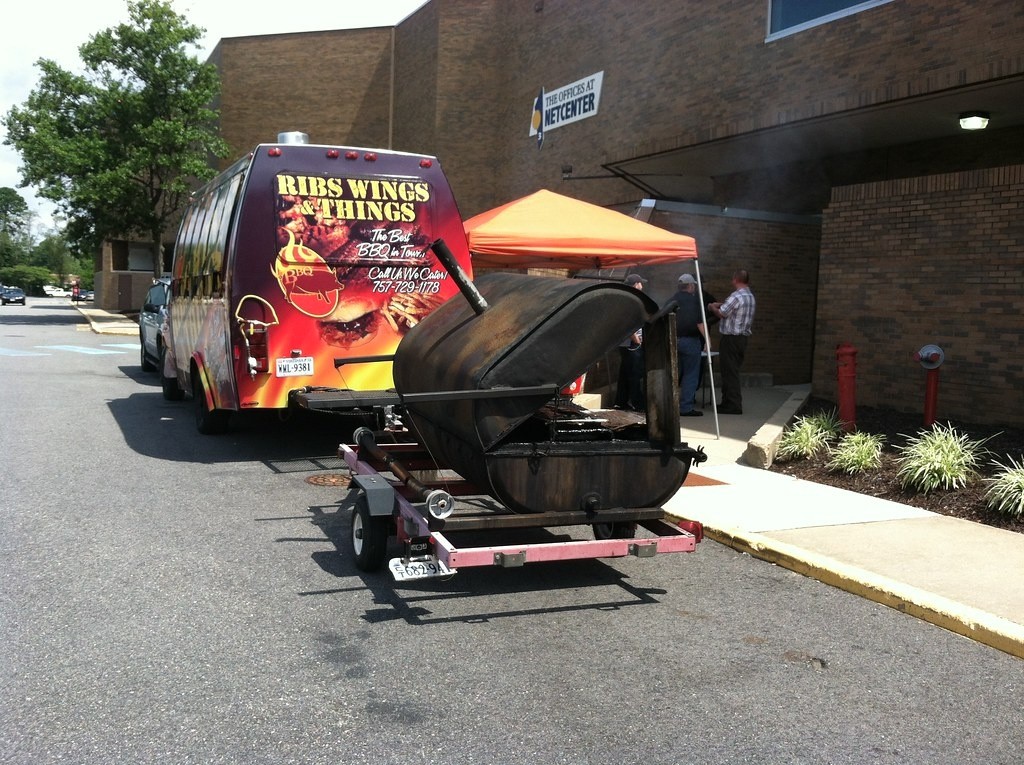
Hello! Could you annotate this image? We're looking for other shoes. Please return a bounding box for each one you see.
[680,410,703,417]
[713,404,742,414]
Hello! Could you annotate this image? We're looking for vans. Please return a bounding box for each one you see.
[162,131,474,437]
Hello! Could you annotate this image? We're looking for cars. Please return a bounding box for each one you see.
[71,289,87,301]
[139,277,182,401]
[86,291,95,301]
[0,288,26,306]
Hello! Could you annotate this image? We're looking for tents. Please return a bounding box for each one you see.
[463,189,721,439]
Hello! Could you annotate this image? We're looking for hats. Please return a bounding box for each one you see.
[624,274,648,286]
[678,273,697,284]
[692,272,707,284]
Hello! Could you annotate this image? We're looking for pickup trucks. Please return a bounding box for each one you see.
[45,288,73,297]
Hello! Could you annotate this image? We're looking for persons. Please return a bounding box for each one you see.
[613,274,649,413]
[707,270,756,415]
[666,272,720,416]
